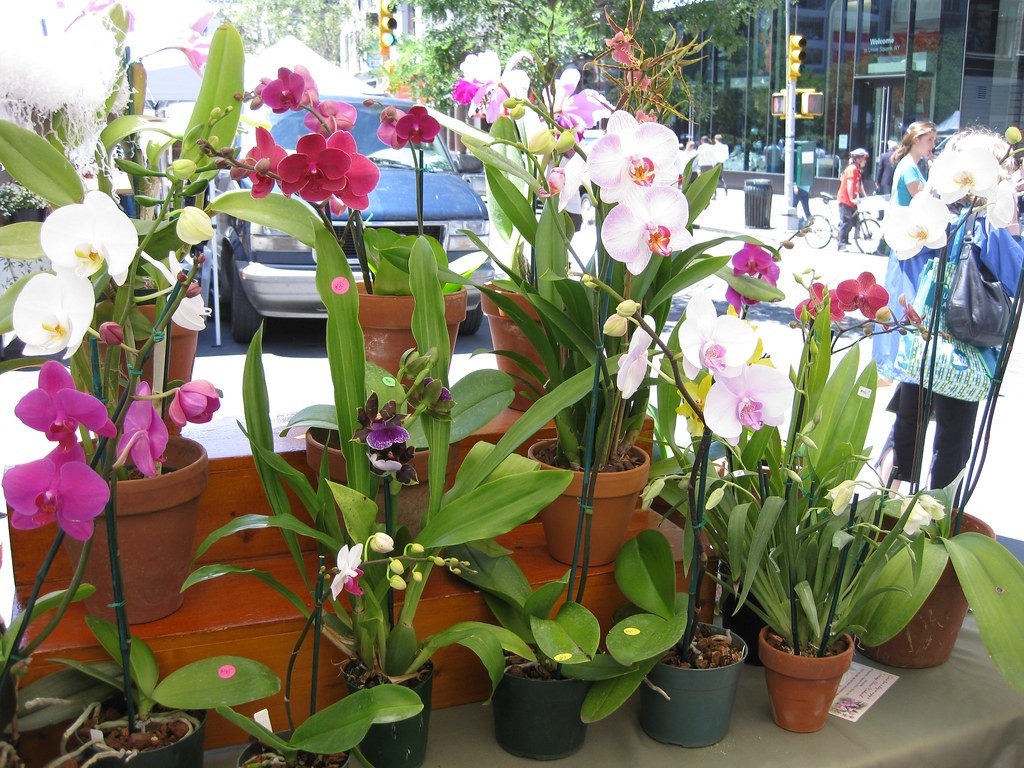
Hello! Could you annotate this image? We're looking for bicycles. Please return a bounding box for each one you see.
[803,192,882,256]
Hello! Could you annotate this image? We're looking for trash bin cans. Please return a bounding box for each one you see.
[744,179,773,229]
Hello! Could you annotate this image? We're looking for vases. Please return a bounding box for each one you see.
[353,283,469,408]
[483,284,567,409]
[529,440,649,570]
[235,724,352,768]
[58,435,207,625]
[338,653,437,768]
[758,621,857,734]
[627,623,743,748]
[75,690,208,768]
[306,428,342,490]
[850,506,993,671]
[492,649,600,762]
[84,281,205,398]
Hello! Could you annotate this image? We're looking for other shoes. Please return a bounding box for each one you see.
[838,246,849,252]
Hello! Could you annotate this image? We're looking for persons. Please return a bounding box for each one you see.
[731,136,840,179]
[877,119,1024,507]
[679,134,729,199]
[837,149,869,251]
[794,182,815,222]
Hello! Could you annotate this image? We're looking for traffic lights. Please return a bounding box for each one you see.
[801,92,824,116]
[789,35,808,80]
[772,93,786,117]
[380,0,397,48]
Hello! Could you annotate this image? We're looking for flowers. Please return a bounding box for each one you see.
[0,22,1024,768]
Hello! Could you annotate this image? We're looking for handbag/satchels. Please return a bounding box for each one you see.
[947,212,1012,348]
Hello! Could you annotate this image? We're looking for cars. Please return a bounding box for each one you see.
[202,94,496,345]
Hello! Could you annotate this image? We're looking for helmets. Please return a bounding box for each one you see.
[850,149,868,158]
